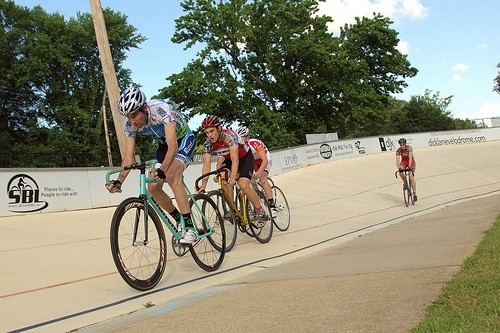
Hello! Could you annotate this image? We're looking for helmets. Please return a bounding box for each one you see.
[117,85,147,116]
[398,138,407,144]
[201,115,221,128]
[235,126,250,137]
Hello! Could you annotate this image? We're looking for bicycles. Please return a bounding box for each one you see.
[103,158,225,293]
[193,164,273,253]
[394,167,417,207]
[223,171,290,238]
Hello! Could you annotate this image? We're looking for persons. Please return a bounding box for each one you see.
[396,139,417,202]
[234,126,279,216]
[191,115,266,220]
[106,86,199,244]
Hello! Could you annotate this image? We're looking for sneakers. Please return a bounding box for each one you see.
[178,229,199,245]
[269,203,276,210]
[413,195,418,201]
[224,210,236,219]
[257,211,268,224]
